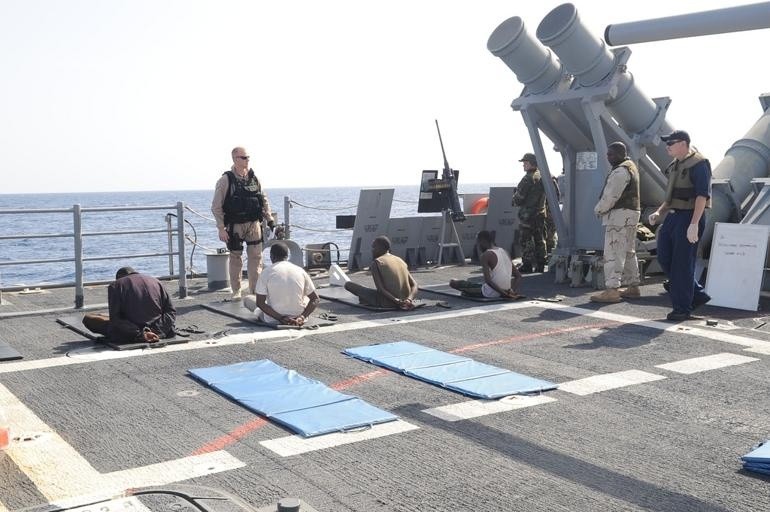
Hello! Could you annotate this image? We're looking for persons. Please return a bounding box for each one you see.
[242,242,320,326]
[590,141,642,301]
[648,128,712,321]
[343,236,418,310]
[82,267,176,342]
[211,146,278,301]
[509,154,548,274]
[545,177,562,266]
[450,230,521,298]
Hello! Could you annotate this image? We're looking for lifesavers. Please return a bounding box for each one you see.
[473,197,490,214]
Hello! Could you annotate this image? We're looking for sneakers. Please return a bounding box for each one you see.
[691,291,713,312]
[230,288,242,302]
[619,284,643,299]
[590,289,623,304]
[664,306,691,321]
[518,263,545,275]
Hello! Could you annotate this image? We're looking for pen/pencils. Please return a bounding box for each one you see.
[754,322,767,329]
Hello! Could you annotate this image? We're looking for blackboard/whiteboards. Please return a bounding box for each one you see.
[705,222,770,311]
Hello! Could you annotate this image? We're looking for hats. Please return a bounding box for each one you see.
[659,129,691,141]
[516,153,537,162]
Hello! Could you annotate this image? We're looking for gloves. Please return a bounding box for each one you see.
[647,211,661,226]
[684,223,700,245]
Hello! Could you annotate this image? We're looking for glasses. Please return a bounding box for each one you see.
[235,154,250,160]
[665,140,686,147]
